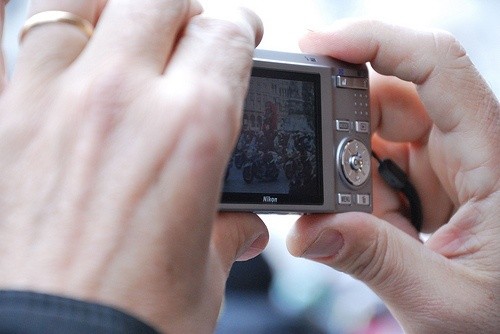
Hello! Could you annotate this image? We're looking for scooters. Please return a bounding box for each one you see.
[236,113,318,184]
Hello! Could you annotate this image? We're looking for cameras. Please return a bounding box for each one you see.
[216,48,374,214]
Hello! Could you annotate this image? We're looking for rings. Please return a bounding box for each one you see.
[18,10,94,44]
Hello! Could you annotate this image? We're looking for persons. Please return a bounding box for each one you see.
[0,0,500,334]
[232,122,320,194]
